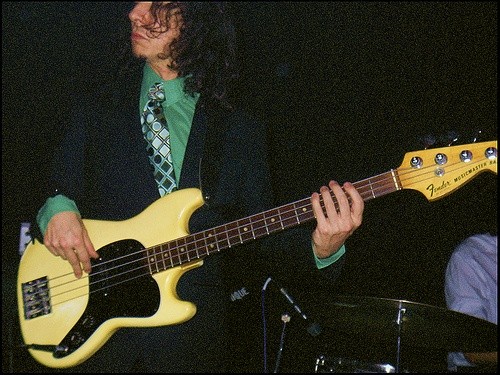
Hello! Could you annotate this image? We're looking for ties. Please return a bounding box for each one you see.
[141,83,189,291]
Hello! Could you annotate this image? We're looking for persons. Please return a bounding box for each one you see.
[24,0,365,375]
[444,184,500,375]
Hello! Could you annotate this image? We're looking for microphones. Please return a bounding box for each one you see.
[274,284,322,339]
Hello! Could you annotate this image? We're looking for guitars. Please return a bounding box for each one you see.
[17,131,497,370]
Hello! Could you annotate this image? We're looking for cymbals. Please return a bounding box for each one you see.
[305,292,497,352]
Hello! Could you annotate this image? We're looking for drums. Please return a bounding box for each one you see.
[309,355,410,374]
